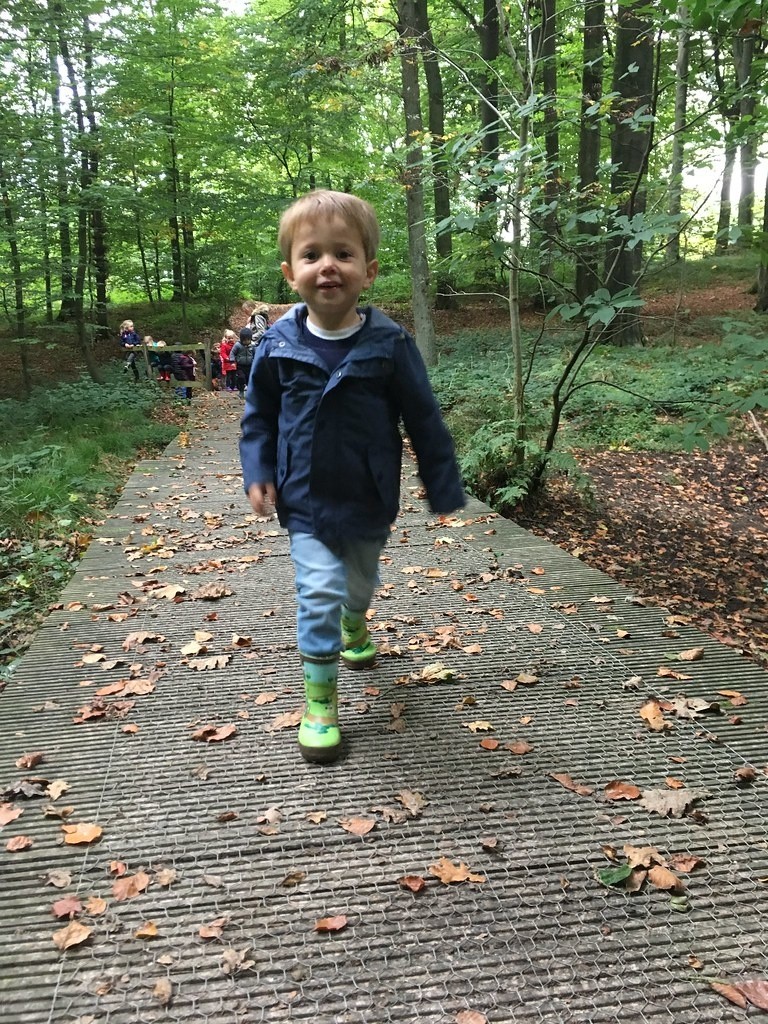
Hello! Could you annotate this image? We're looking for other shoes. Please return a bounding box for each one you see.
[225,386,238,392]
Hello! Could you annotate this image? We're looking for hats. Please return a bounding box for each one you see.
[240,328,252,346]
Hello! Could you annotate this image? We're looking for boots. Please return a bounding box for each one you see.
[339,602,377,668]
[297,653,340,762]
[157,372,171,381]
[123,362,131,374]
[133,370,139,383]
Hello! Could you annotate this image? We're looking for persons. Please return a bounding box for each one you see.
[180,350,197,399]
[242,299,270,342]
[198,342,226,391]
[220,330,238,391]
[230,327,256,397]
[238,189,468,761]
[149,340,178,381]
[136,336,161,380]
[119,320,142,380]
[171,342,188,399]
